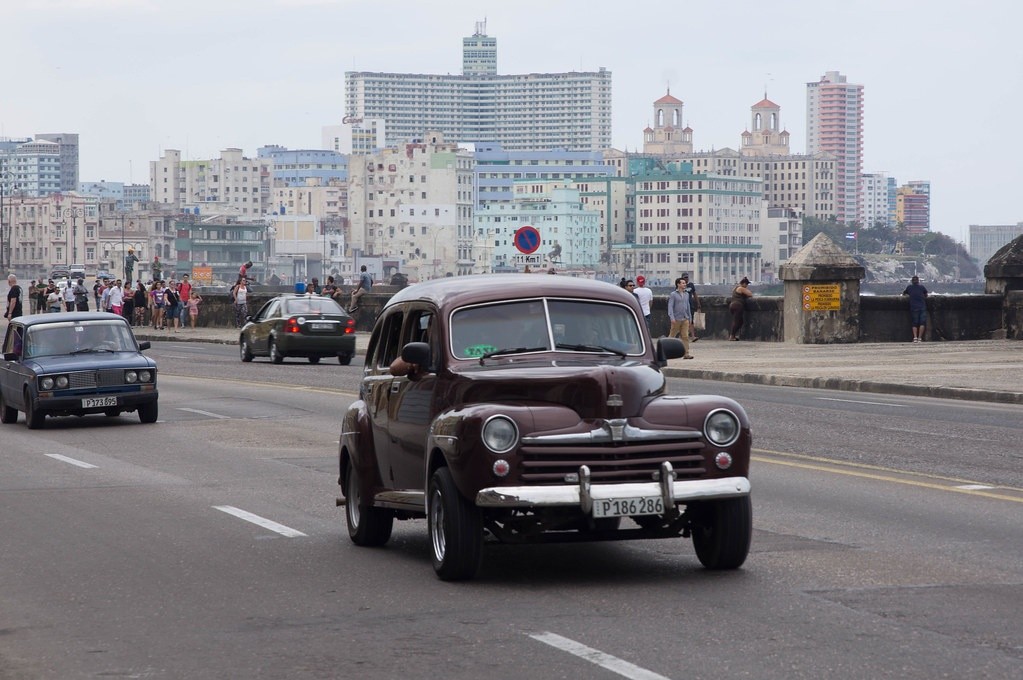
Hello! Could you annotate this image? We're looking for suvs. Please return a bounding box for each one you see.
[50,264,70,280]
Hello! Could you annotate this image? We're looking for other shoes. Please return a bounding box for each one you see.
[347,306,359,313]
[692,336,698,342]
[683,355,694,359]
[729,337,738,341]
[135,323,196,333]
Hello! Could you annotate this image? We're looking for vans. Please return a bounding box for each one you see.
[69,263,86,280]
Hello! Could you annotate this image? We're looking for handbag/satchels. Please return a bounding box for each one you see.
[693,308,706,330]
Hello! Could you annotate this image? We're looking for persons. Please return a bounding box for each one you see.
[330,267,344,285]
[320,275,342,299]
[94,277,168,330]
[728,276,753,341]
[348,264,373,314]
[233,276,248,329]
[28,278,89,315]
[164,273,202,334]
[151,257,162,281]
[4,274,23,322]
[682,273,701,342]
[525,265,531,273]
[548,267,556,273]
[303,277,322,298]
[390,312,465,377]
[230,260,256,293]
[668,278,694,359]
[619,275,653,338]
[903,276,928,343]
[125,247,139,287]
[80,325,122,353]
[561,312,639,354]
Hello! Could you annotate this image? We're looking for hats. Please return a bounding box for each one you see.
[637,276,645,285]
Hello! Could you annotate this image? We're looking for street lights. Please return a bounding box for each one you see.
[427,227,445,273]
[114,213,134,279]
[61,207,87,263]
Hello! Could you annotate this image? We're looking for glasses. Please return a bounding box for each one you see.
[627,285,635,287]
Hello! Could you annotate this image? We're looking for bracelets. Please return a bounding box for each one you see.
[8,313,12,314]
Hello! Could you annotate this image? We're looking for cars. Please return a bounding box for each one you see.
[0,311,159,430]
[239,293,357,366]
[335,272,755,584]
[95,268,117,280]
